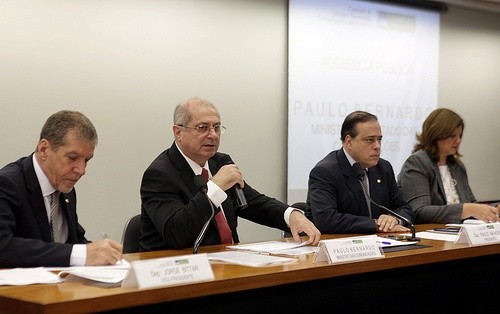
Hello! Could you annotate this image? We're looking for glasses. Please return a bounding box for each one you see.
[177,123,226,136]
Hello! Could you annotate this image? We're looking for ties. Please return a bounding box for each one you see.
[51,191,60,243]
[362,169,372,219]
[202,168,232,245]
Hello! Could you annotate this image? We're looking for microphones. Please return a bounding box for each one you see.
[221,155,248,210]
[353,162,420,241]
[193,175,214,255]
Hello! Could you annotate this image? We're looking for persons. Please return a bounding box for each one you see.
[138,99,321,252]
[300,111,414,236]
[397,108,500,224]
[0,109,122,269]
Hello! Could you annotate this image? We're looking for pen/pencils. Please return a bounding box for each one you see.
[102,234,124,265]
[376,241,390,244]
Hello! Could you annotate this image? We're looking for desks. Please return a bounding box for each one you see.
[0,223,500,314]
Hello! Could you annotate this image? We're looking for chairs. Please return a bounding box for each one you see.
[122,202,306,253]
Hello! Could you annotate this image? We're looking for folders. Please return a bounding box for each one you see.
[434,226,462,232]
